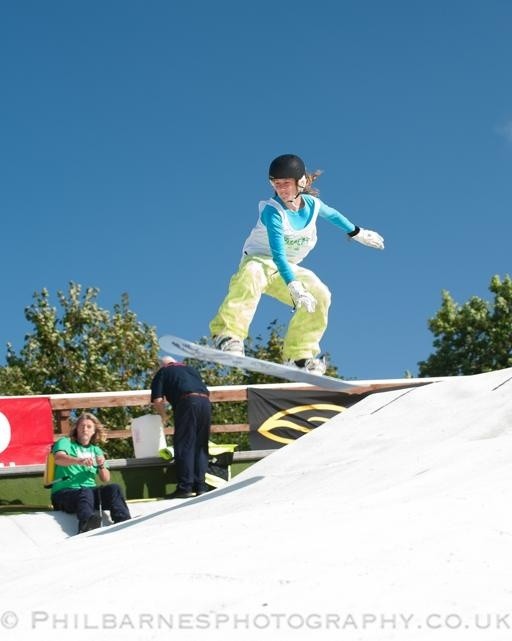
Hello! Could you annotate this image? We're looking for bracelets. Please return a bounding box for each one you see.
[97,464,104,470]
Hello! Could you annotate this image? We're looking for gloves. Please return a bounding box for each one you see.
[351,226,385,251]
[287,279,318,314]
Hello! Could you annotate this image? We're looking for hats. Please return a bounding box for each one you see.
[161,355,176,364]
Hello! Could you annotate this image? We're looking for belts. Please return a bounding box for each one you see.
[179,392,208,398]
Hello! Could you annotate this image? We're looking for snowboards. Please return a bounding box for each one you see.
[159,335,358,392]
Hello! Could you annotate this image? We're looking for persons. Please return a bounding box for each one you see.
[51,412,132,534]
[149,356,213,497]
[208,153,385,375]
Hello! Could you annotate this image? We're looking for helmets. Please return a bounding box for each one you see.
[270,155,309,204]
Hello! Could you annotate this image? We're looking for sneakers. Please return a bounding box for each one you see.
[78,512,101,532]
[216,333,244,351]
[284,357,327,371]
[175,485,197,497]
[197,489,206,494]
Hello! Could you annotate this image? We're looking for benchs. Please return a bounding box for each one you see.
[1,450,235,490]
